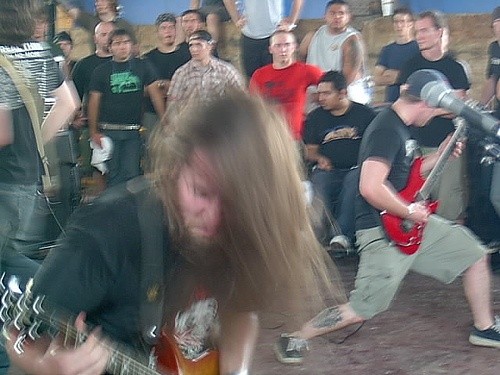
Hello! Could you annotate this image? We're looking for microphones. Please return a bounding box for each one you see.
[404,68,500,138]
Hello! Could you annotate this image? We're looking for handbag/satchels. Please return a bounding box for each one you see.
[41,158,62,193]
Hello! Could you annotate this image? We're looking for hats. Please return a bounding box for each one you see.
[188,30,212,42]
[404,69,451,97]
[155,13,176,25]
[50,31,72,43]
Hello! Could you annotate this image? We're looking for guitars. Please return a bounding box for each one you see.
[378,115,465,256]
[1,272,225,375]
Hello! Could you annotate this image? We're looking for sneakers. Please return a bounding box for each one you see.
[469,324,500,348]
[271,331,304,363]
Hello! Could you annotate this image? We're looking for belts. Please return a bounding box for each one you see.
[97,122,141,130]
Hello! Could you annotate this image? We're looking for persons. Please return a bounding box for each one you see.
[7,87,350,375]
[272,69,500,364]
[0,0,244,293]
[223,0,500,255]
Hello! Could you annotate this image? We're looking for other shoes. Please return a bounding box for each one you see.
[331,236,349,258]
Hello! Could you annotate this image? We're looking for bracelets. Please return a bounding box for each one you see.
[406,205,415,219]
